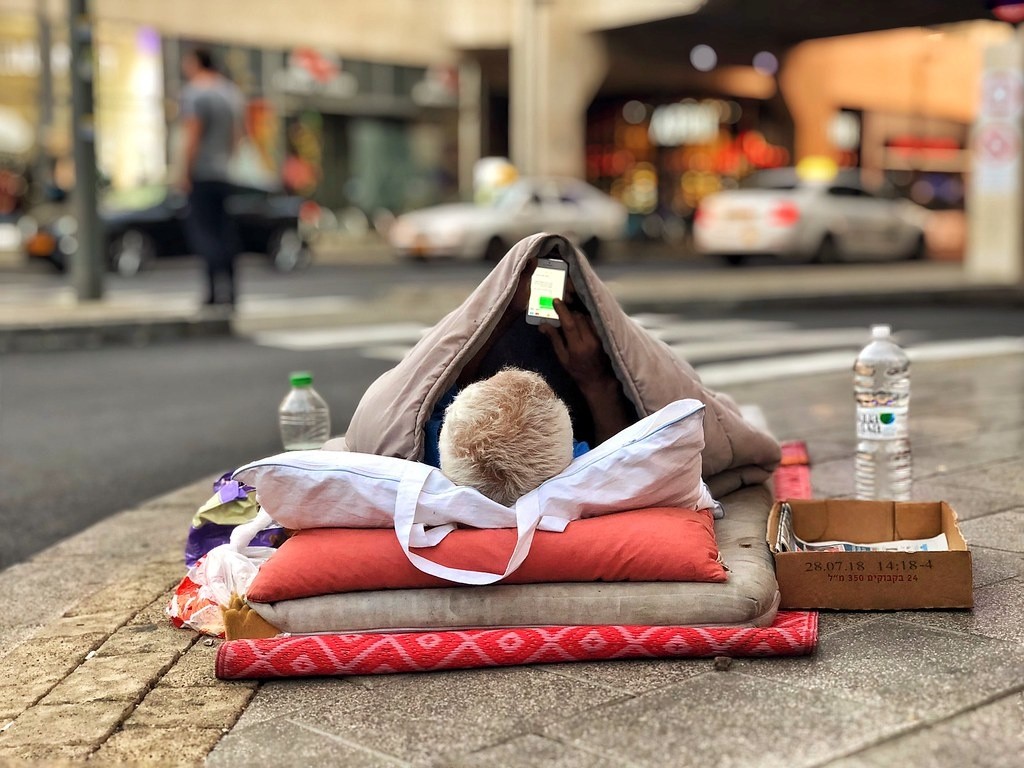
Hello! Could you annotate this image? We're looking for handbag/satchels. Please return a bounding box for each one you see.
[227,135,276,191]
[167,506,278,639]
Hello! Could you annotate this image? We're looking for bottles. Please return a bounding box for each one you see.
[275,371,333,453]
[850,322,913,502]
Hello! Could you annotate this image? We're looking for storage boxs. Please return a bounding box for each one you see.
[765,500,974,610]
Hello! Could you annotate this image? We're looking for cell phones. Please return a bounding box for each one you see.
[526,258,568,328]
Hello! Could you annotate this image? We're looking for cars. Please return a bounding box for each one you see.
[691,164,927,270]
[387,167,629,266]
[19,178,323,280]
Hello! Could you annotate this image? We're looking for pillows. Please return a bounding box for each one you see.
[230,399,724,533]
[245,508,728,602]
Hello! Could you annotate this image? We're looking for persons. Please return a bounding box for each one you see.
[424,258,629,508]
[177,49,252,321]
